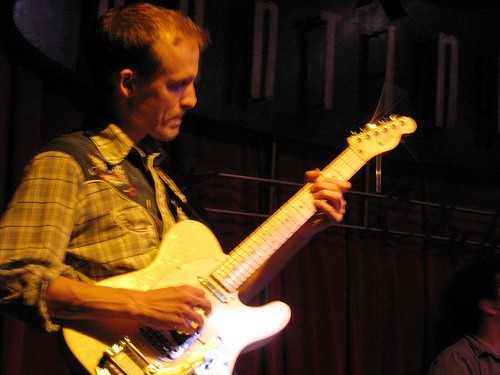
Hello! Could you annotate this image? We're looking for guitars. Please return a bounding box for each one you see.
[61,113,417,375]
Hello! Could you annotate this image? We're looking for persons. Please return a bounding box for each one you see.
[429,263,500,375]
[1,2,351,375]
